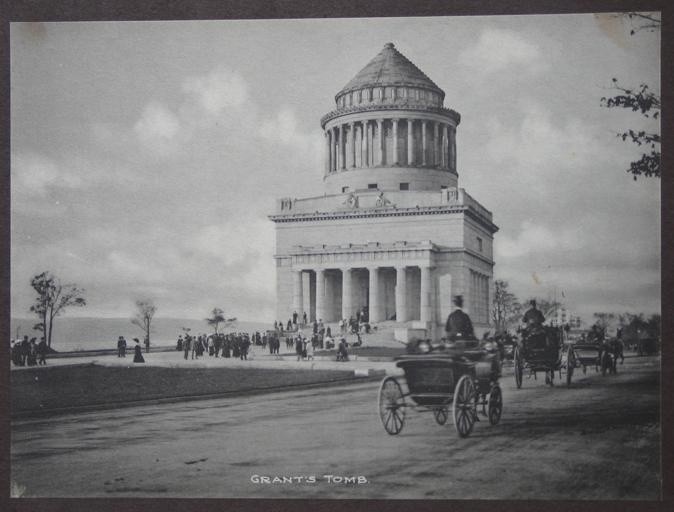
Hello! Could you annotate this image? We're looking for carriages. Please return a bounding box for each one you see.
[498,335,519,378]
[378,331,505,439]
[513,323,575,390]
[571,327,625,376]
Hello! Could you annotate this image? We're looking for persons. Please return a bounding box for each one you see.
[587,324,603,341]
[446,294,476,343]
[176,306,371,362]
[522,300,545,332]
[482,328,520,364]
[9,334,49,366]
[117,333,150,363]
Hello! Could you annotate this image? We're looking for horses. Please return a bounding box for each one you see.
[545,324,565,387]
[605,327,627,376]
[472,331,503,422]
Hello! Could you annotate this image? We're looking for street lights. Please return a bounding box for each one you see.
[637,325,641,356]
